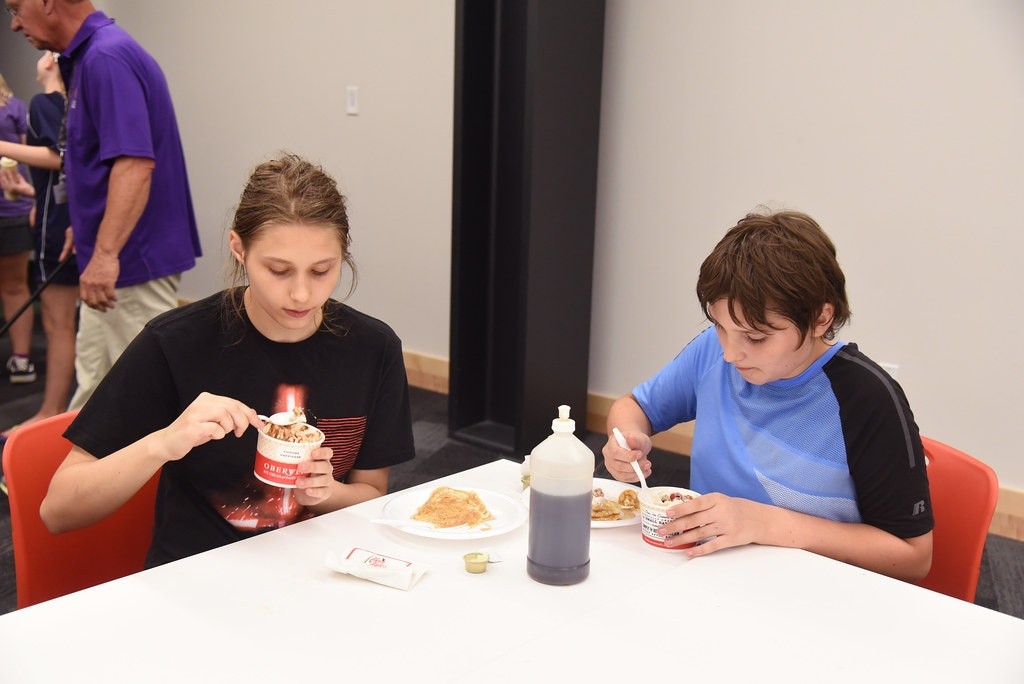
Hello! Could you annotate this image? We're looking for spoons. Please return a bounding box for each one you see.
[258,412,297,426]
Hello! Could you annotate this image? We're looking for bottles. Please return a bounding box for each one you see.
[526,404,595,585]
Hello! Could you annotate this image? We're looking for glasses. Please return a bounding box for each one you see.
[4,4,21,16]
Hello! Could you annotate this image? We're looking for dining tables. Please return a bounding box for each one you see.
[0,459,1024,684]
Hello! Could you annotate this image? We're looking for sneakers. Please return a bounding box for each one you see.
[6,356,36,383]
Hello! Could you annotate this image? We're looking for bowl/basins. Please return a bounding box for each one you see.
[254,422,326,489]
[638,486,703,552]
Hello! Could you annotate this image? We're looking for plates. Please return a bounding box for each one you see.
[383,483,527,540]
[589,477,645,529]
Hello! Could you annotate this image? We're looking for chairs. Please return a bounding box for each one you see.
[1,408,162,613]
[909,436,999,603]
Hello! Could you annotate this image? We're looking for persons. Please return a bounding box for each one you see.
[40,152,416,570]
[0,74,38,382]
[0,50,80,444]
[0,0,203,413]
[602,210,936,582]
